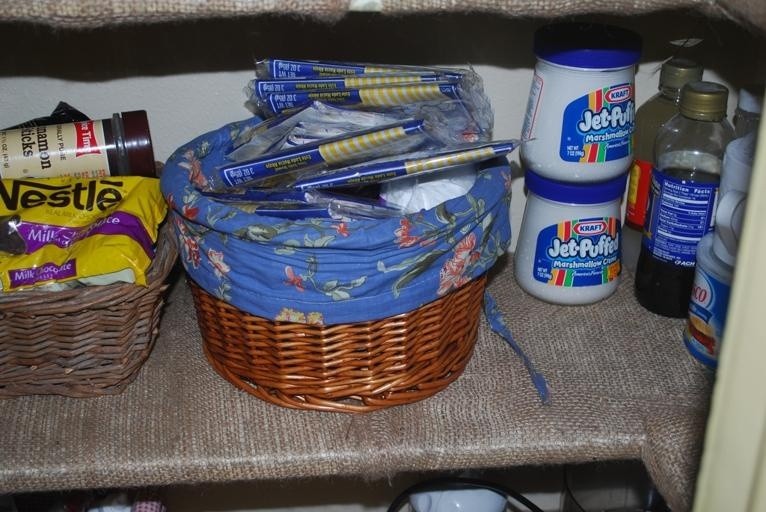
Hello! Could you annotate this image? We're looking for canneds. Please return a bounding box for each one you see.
[0,109,157,179]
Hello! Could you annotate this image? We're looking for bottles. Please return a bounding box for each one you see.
[624,58,764,320]
[0,110,158,182]
